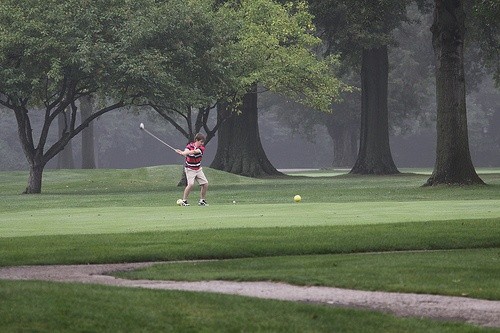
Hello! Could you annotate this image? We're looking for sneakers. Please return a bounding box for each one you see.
[198,200,209,206]
[181,200,190,206]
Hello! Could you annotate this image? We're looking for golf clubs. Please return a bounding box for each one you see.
[140,122,187,157]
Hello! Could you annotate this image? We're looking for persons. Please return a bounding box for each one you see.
[176,133,209,207]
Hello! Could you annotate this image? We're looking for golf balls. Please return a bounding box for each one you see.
[233,200,236,203]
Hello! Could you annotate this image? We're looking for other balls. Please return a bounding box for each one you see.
[294,194,301,202]
